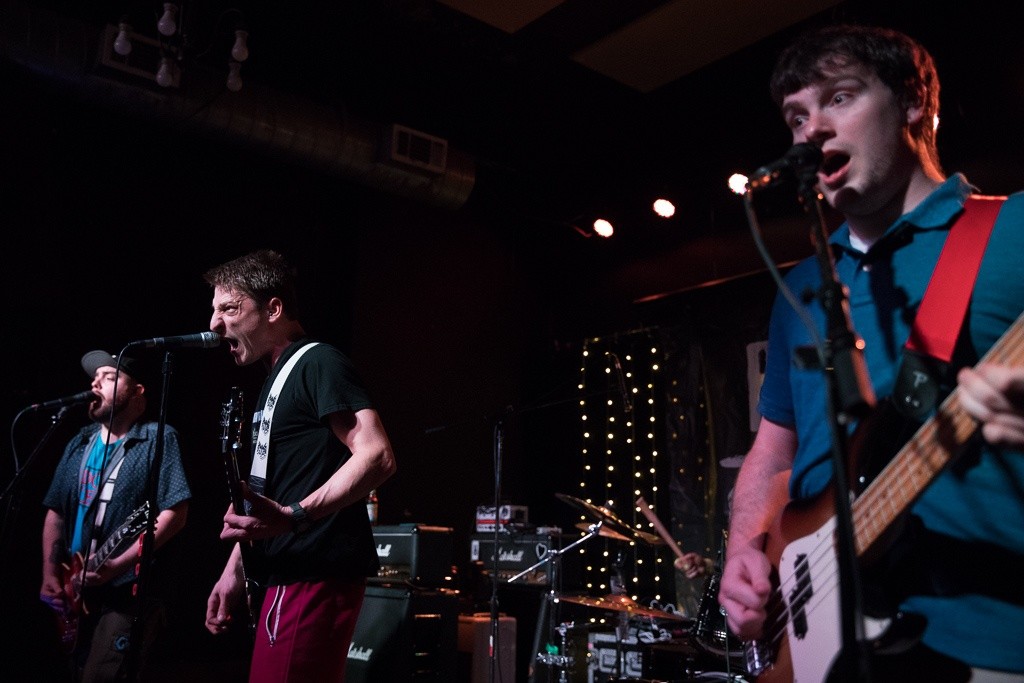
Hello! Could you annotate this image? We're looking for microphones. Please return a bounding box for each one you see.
[746,142,825,191]
[33,391,97,411]
[138,332,222,348]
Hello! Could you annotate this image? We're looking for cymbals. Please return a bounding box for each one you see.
[556,494,683,547]
[557,594,695,624]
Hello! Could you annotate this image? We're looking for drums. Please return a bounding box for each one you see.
[690,575,744,659]
[661,671,749,683]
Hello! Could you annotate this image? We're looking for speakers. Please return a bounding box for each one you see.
[470,532,562,683]
[345,585,463,683]
[458,615,516,683]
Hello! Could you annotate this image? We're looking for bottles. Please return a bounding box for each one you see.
[367,489,378,526]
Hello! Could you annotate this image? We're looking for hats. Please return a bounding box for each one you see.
[80,350,154,394]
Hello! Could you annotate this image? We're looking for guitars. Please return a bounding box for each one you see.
[217,386,264,632]
[739,309,1024,683]
[55,500,160,641]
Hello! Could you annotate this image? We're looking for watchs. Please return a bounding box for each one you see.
[288,501,310,533]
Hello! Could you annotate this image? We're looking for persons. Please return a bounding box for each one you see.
[40,350,192,682]
[718,27,1023,683]
[205,250,397,683]
[674,552,716,579]
